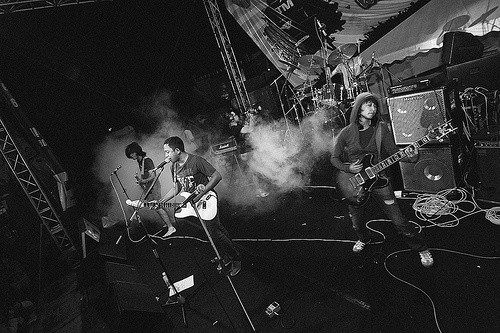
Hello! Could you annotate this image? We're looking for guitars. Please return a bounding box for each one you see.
[332,118,458,208]
[125,191,218,221]
[133,172,146,190]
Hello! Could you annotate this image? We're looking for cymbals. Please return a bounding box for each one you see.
[298,54,326,75]
[293,81,318,91]
[328,43,357,67]
[289,92,316,100]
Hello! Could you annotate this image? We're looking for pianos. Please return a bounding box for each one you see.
[209,135,255,190]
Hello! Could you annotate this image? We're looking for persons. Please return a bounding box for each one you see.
[125,142,176,237]
[155,136,242,276]
[330,92,434,267]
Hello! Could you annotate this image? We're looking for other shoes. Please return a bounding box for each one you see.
[162,224,167,228]
[162,228,177,238]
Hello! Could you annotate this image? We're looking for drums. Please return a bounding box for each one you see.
[322,83,346,106]
[314,101,352,128]
[346,85,367,100]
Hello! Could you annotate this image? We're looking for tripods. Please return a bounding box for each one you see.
[115,165,209,328]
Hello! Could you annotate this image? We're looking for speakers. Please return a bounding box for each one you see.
[441,32,484,66]
[386,86,500,196]
[71,240,174,333]
[217,149,254,188]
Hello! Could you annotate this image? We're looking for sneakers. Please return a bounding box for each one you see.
[230,260,242,276]
[217,260,232,270]
[419,248,433,268]
[352,238,372,253]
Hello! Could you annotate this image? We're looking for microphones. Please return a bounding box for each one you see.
[315,18,327,36]
[111,165,121,175]
[293,35,310,49]
[370,53,375,66]
[152,158,172,172]
[175,190,201,213]
